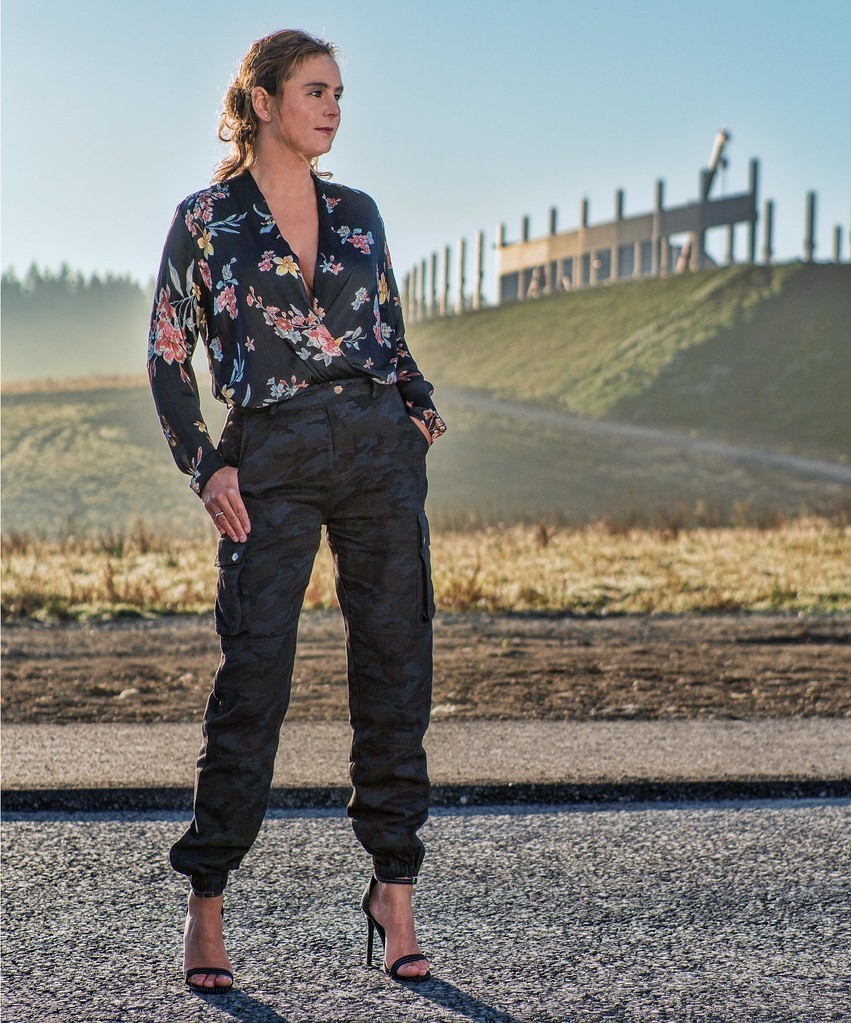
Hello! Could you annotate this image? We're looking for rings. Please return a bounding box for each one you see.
[215,511,224,518]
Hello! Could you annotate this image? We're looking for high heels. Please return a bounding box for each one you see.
[183,904,234,994]
[360,873,431,982]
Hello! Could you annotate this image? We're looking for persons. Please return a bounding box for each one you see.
[147,29,448,996]
